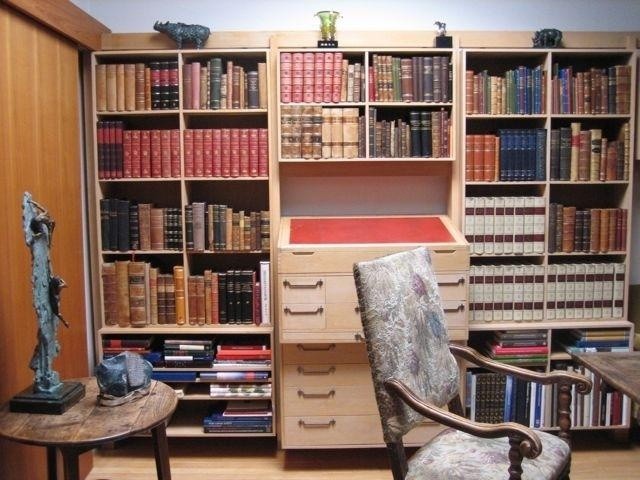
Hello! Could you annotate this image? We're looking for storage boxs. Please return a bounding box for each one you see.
[279,345,465,451]
[278,215,470,338]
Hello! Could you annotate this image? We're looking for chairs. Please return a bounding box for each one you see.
[353,246,592,480]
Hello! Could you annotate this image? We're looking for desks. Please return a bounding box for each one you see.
[0,376,179,480]
[571,351,640,405]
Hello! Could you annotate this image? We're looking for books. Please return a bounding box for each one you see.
[96,56,268,112]
[97,121,269,178]
[100,199,270,252]
[470,264,625,322]
[102,338,274,433]
[369,54,454,159]
[281,52,367,160]
[466,65,630,116]
[466,122,630,182]
[465,196,627,254]
[101,261,271,327]
[465,328,629,428]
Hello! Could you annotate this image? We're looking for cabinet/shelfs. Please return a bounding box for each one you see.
[90,48,636,450]
